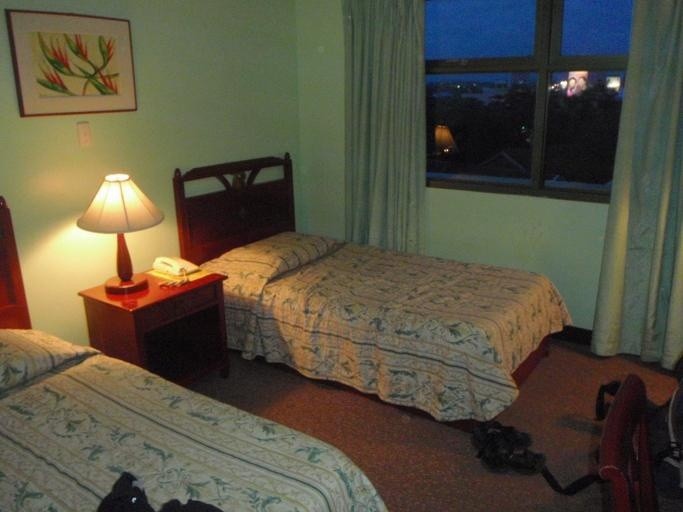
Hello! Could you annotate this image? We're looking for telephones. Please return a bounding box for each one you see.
[152,256,200,276]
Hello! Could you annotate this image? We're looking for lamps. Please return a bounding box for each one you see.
[74,172,165,295]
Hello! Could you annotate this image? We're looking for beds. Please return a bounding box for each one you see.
[0,193,357,512]
[171,152,553,437]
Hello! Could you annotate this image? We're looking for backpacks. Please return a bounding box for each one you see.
[649,381,683,501]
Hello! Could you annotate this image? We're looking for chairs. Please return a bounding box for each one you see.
[597,373,658,511]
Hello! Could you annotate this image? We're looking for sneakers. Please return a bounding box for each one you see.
[472,420,533,452]
[481,447,547,475]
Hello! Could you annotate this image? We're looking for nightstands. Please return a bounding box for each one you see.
[75,269,229,382]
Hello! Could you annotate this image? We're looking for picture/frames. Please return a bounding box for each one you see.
[4,8,137,118]
